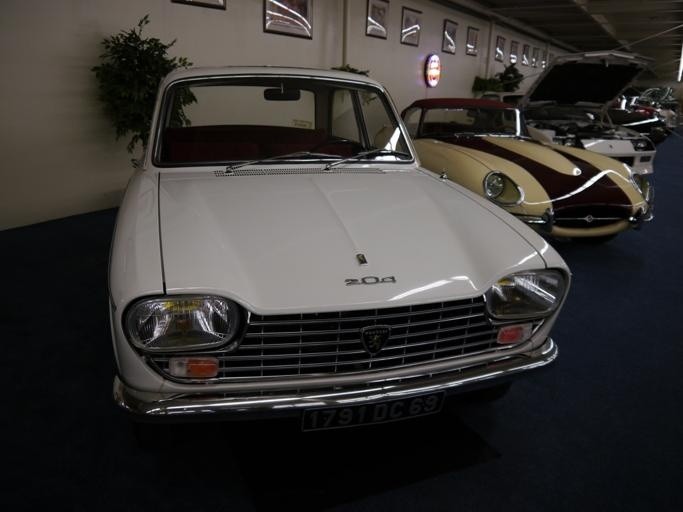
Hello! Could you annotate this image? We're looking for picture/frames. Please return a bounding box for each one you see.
[365,1,389,39]
[495,35,506,63]
[171,2,227,11]
[466,25,480,57]
[521,44,531,66]
[262,2,313,39]
[400,6,423,47]
[441,18,459,55]
[509,40,519,64]
[532,46,540,67]
[541,50,555,68]
